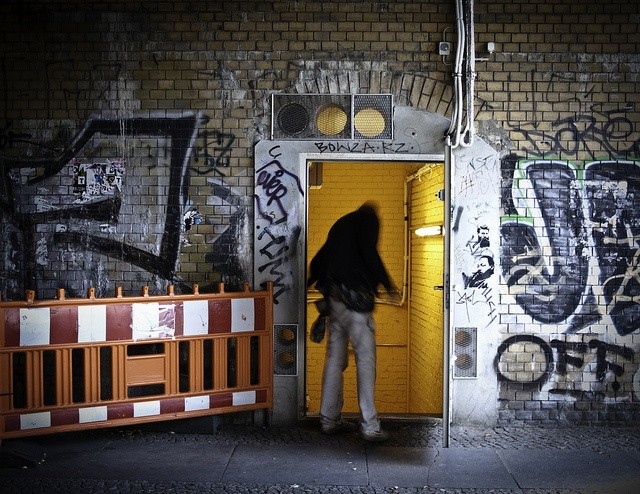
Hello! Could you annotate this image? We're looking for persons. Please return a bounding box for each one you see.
[308,201,399,441]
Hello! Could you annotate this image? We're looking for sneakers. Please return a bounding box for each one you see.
[363,430,400,441]
[323,420,360,431]
